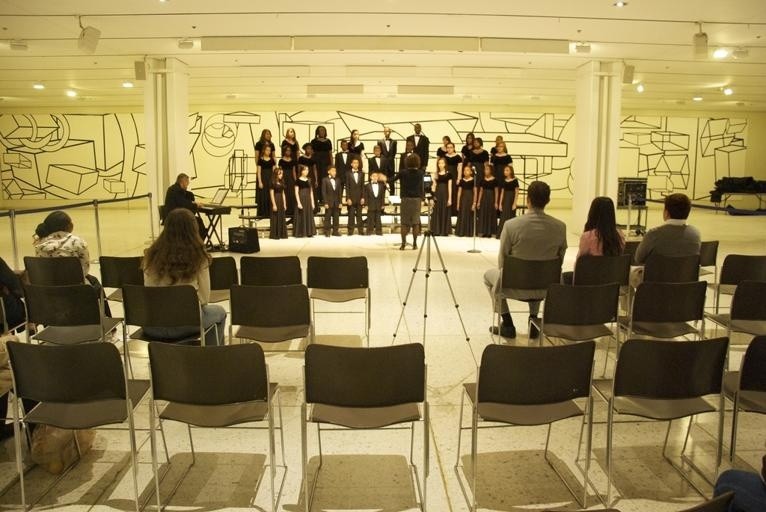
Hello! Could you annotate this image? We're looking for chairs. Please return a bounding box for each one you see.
[576,336,727,512]
[453,341,596,512]
[681,333,765,484]
[6,340,140,512]
[681,490,734,511]
[149,342,288,510]
[301,343,428,511]
[488,240,765,341]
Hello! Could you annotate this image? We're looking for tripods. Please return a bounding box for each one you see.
[392,232,479,375]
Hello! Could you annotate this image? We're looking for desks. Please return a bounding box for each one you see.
[615,206,648,239]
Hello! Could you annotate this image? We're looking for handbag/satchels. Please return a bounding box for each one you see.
[228,225,260,253]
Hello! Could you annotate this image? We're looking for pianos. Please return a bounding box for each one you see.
[196,204,232,215]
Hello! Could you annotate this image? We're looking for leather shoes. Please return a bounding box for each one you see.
[530,325,540,338]
[490,324,515,338]
[2,422,21,438]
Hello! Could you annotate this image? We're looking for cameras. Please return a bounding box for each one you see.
[423,174,432,193]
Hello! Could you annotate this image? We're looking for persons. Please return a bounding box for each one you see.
[0,258,36,450]
[368,146,388,215]
[490,143,512,188]
[344,157,365,236]
[140,208,227,347]
[490,135,508,155]
[455,163,477,237]
[298,144,321,215]
[256,144,277,220]
[254,130,275,165]
[165,174,207,242]
[377,127,397,195]
[442,143,463,216]
[429,157,453,236]
[495,166,520,241]
[292,164,316,237]
[476,162,499,238]
[311,126,333,205]
[400,141,423,168]
[467,137,490,189]
[281,128,299,161]
[461,134,475,163]
[33,211,117,332]
[347,129,364,160]
[268,168,288,240]
[437,136,451,157]
[379,154,426,250]
[335,140,355,192]
[406,124,430,166]
[561,197,626,286]
[365,170,384,236]
[277,145,297,214]
[321,165,342,237]
[483,181,567,339]
[621,193,702,315]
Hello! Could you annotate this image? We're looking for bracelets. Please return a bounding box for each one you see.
[472,203,476,205]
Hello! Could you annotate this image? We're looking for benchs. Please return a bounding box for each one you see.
[242,179,529,233]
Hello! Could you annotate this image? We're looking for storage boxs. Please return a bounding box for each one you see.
[617,177,647,207]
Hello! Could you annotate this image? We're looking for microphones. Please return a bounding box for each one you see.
[470,165,480,174]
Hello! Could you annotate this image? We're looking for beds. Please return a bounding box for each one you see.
[710,189,766,215]
